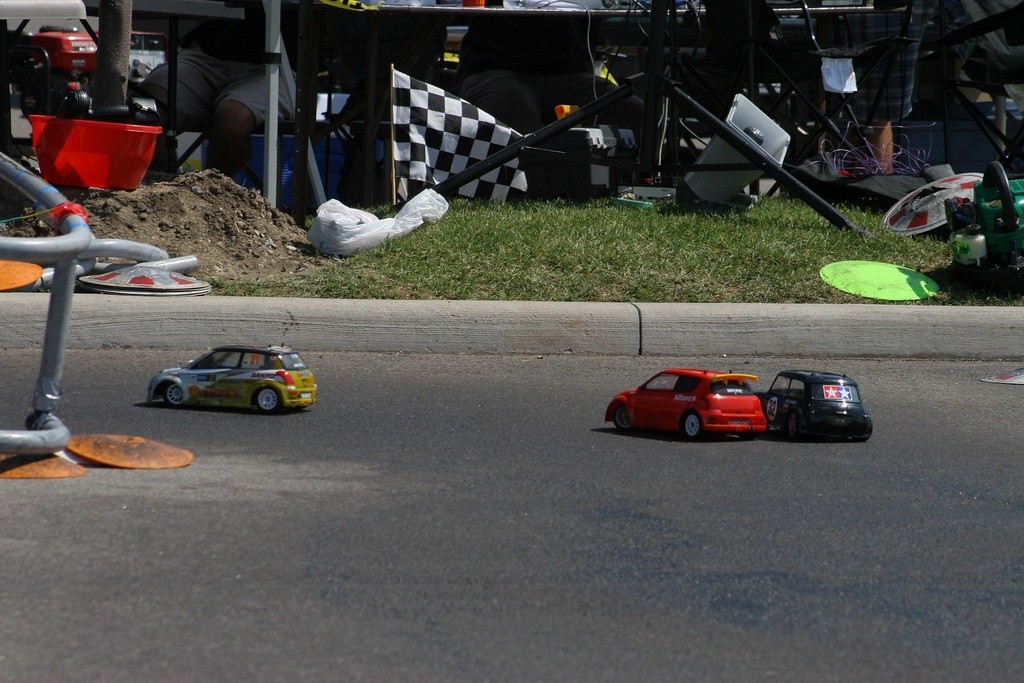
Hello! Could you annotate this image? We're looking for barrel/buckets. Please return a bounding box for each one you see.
[28,113,162,190]
[200,132,393,215]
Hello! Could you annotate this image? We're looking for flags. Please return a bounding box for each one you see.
[390,68,528,204]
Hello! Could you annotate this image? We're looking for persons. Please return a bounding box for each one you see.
[127,0,321,181]
[442,15,644,202]
[827,1,977,156]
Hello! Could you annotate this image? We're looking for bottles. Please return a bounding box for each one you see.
[61,82,92,119]
[951,223,987,264]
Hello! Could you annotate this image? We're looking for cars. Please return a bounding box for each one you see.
[763,371,873,442]
[605,370,767,443]
[148,345,319,414]
[18,25,170,116]
